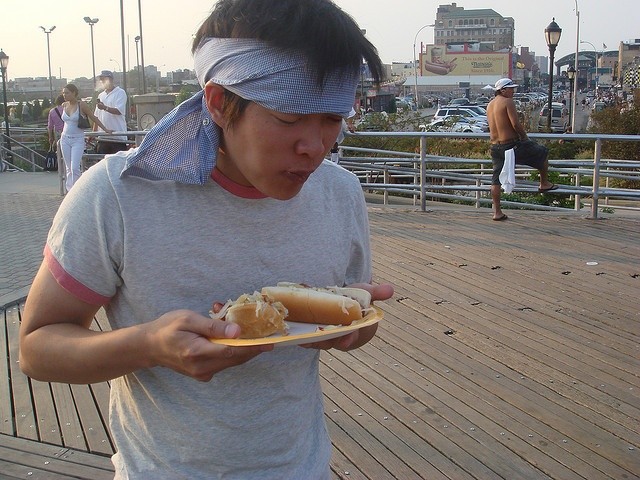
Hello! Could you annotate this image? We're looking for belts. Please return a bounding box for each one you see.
[490,137,519,145]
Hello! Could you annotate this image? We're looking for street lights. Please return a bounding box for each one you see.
[413,21,444,112]
[0,47,13,172]
[83,16,99,90]
[567,66,576,133]
[134,35,142,95]
[544,17,562,143]
[579,39,599,100]
[38,25,57,104]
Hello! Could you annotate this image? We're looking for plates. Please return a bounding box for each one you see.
[206,302,385,347]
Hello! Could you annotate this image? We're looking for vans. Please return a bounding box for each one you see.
[538,101,570,132]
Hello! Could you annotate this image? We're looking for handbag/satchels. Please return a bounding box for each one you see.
[44,144,58,171]
[78,100,91,128]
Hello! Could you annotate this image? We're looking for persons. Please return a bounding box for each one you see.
[19,0,395,480]
[48,94,66,181]
[9,105,17,119]
[87,71,128,163]
[60,84,116,194]
[485,77,560,221]
[21,103,30,123]
[427,96,441,109]
[580,97,598,111]
[330,106,357,165]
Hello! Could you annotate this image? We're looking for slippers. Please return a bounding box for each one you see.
[539,183,559,193]
[492,214,507,221]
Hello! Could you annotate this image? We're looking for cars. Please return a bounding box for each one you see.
[403,97,416,107]
[395,97,408,108]
[449,98,471,106]
[419,115,484,134]
[446,97,453,106]
[476,97,490,105]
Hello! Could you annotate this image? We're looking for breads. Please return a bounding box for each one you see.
[259,280,376,326]
[210,289,291,340]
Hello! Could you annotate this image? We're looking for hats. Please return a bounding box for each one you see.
[99,71,114,80]
[495,77,519,91]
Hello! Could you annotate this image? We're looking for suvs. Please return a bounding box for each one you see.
[440,106,487,116]
[513,97,533,109]
[431,108,488,131]
